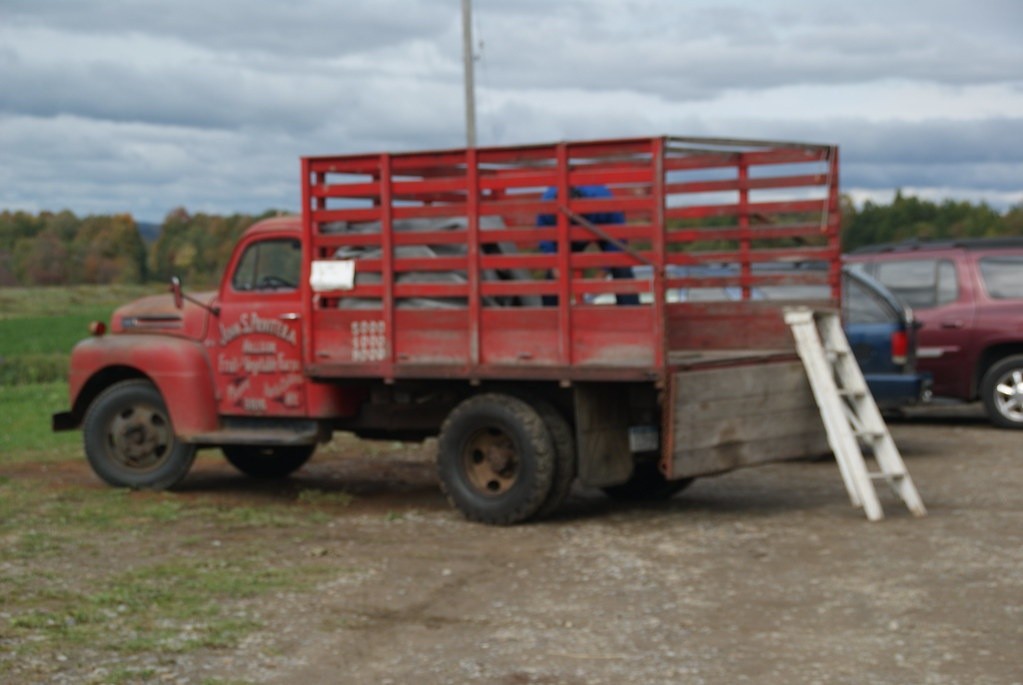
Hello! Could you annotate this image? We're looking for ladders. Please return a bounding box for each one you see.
[782,305,928,521]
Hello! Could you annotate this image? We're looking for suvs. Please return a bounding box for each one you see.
[570,238,1023,430]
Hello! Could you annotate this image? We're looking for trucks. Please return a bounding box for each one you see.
[51,135,843,524]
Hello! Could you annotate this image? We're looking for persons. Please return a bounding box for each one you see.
[535,184,640,306]
